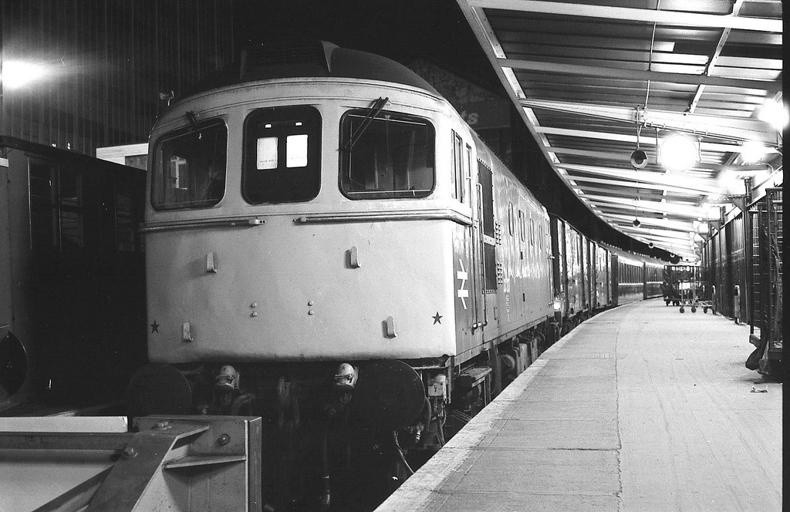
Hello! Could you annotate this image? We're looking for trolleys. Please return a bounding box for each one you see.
[673,278,716,314]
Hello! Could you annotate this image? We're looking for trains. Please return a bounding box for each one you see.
[138,76,705,508]
